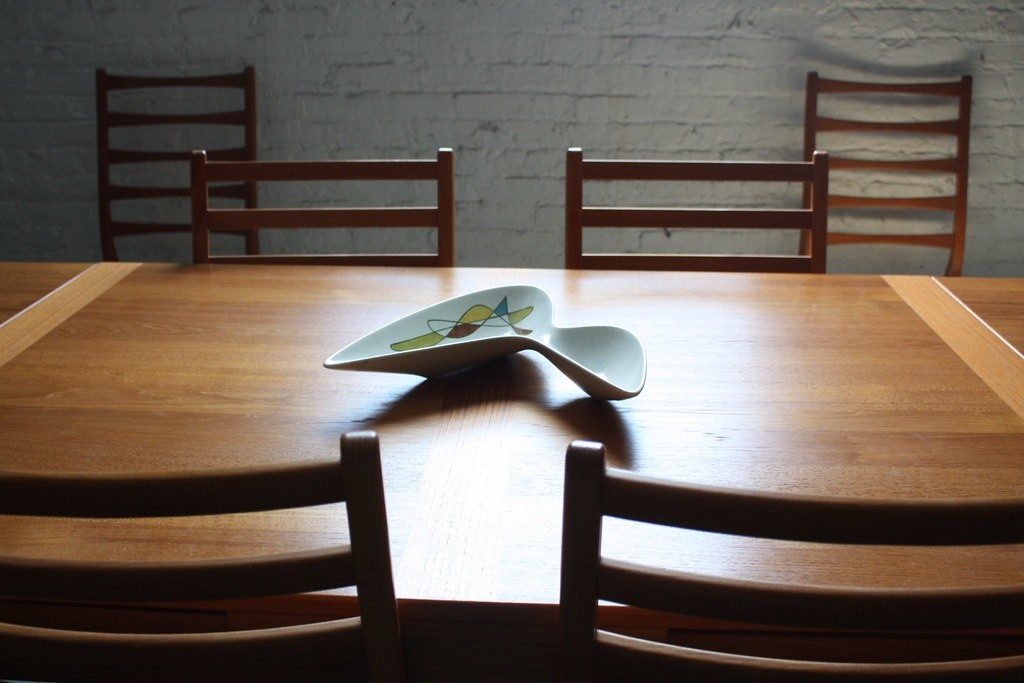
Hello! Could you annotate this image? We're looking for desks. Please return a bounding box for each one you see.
[0,259,1024,682]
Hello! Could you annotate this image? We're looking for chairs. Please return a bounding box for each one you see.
[800,72,973,277]
[556,440,1024,682]
[564,147,829,274]
[1,429,406,683]
[95,65,260,264]
[190,148,454,268]
